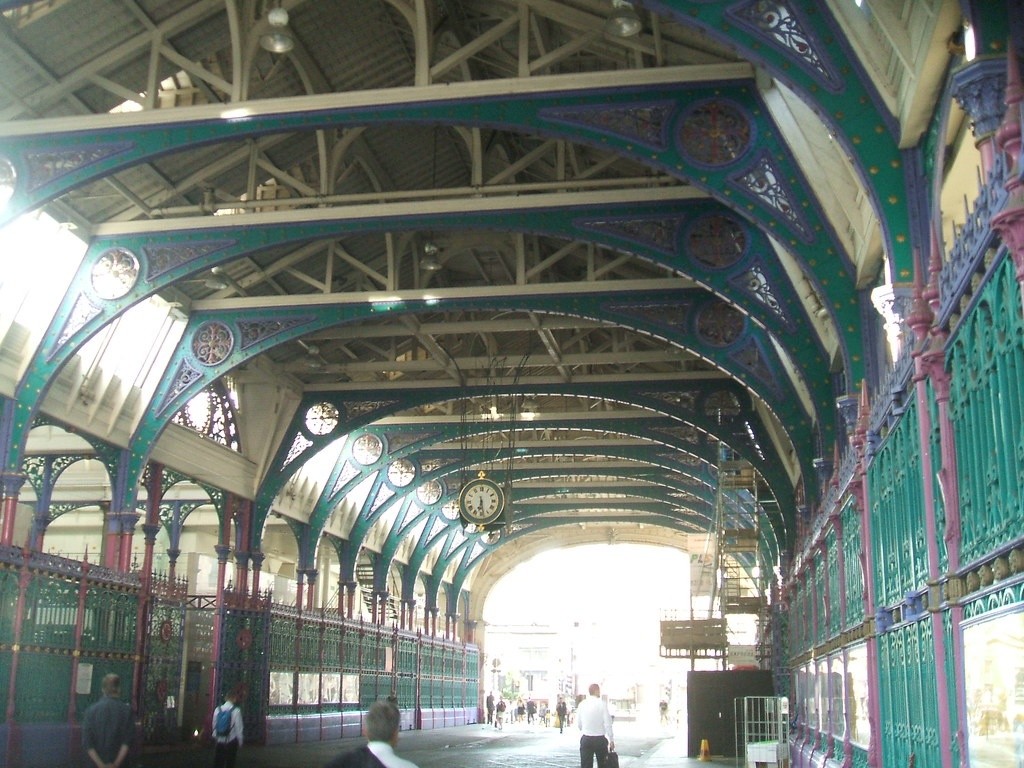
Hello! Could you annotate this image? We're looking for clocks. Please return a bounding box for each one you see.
[458,470,505,526]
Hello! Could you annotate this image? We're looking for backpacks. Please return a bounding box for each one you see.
[213,704,237,735]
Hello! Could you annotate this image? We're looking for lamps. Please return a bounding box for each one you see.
[602,0,644,38]
[259,0,296,54]
[418,127,444,271]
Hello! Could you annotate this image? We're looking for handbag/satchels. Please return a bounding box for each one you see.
[554,716,561,728]
[604,749,619,768]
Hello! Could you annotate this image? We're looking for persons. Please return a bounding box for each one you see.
[82,674,135,768]
[576,684,615,768]
[211,690,243,768]
[386,690,398,705]
[325,701,420,768]
[486,691,571,734]
[659,699,668,722]
